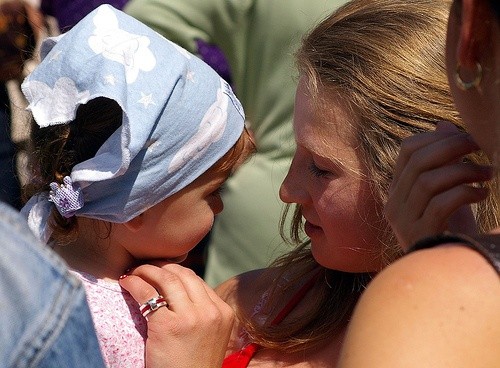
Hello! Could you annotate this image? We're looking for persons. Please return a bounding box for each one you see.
[119,0,500,368]
[19,4,259,368]
[336,0,500,368]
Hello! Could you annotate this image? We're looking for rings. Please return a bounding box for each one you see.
[140,295,167,317]
